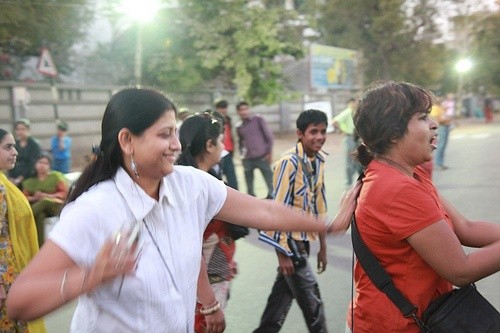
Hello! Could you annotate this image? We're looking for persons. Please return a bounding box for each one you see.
[0,80,500,333]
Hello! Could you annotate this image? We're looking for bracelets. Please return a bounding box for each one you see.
[199,301,220,315]
[60,268,68,302]
[80,265,92,293]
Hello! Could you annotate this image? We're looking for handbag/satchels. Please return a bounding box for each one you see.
[421,285,500,333]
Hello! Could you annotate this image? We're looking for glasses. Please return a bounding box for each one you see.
[203,111,218,124]
